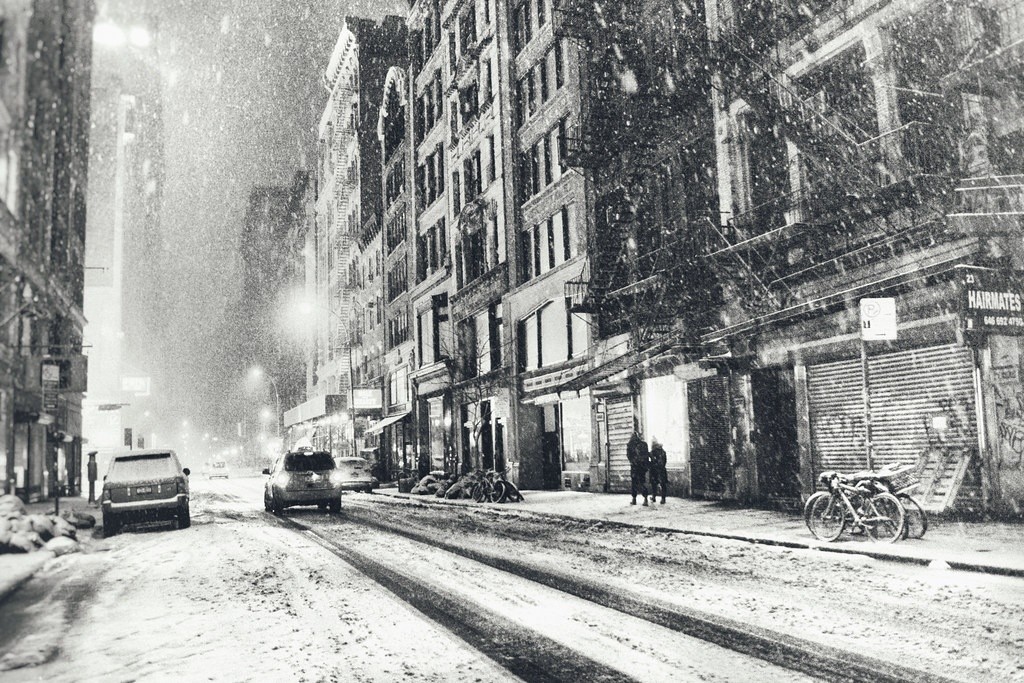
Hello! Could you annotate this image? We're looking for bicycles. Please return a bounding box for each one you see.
[804,472,927,543]
[471,468,525,504]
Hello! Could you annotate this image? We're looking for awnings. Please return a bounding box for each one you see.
[365,417,411,435]
[524,377,589,406]
[553,336,674,395]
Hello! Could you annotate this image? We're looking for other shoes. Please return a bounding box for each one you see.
[660,500,665,503]
[630,502,637,505]
[650,497,656,501]
[642,503,648,506]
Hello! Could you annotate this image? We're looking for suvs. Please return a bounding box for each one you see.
[101,449,190,536]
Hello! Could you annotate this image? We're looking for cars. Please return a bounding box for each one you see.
[262,446,341,516]
[203,461,228,479]
[334,456,372,493]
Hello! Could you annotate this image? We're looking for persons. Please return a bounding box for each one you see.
[626,429,668,508]
